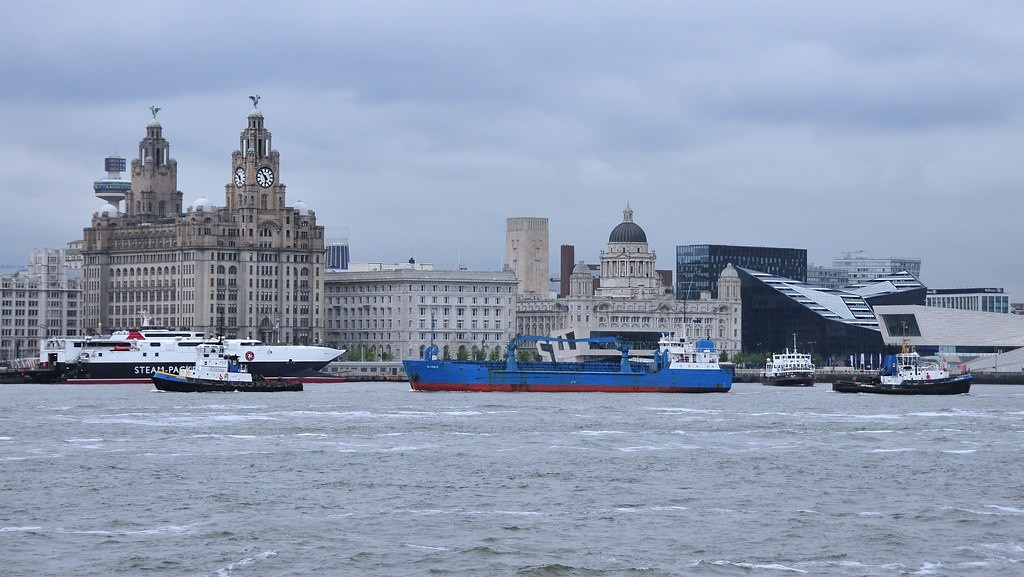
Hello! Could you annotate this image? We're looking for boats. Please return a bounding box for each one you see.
[760,333,817,387]
[0,306,349,384]
[149,304,304,393]
[400,293,737,393]
[831,320,975,396]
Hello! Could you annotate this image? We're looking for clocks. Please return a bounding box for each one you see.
[256,167,274,188]
[234,167,246,188]
